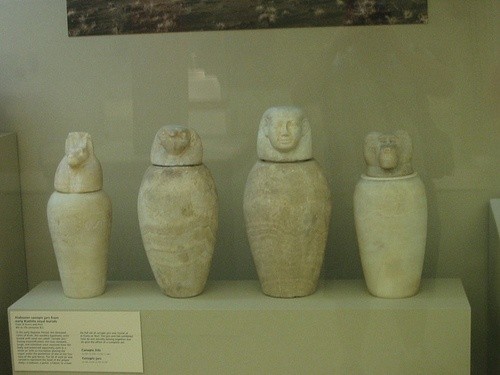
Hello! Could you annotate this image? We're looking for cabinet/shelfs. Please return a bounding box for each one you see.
[7,274,473,375]
